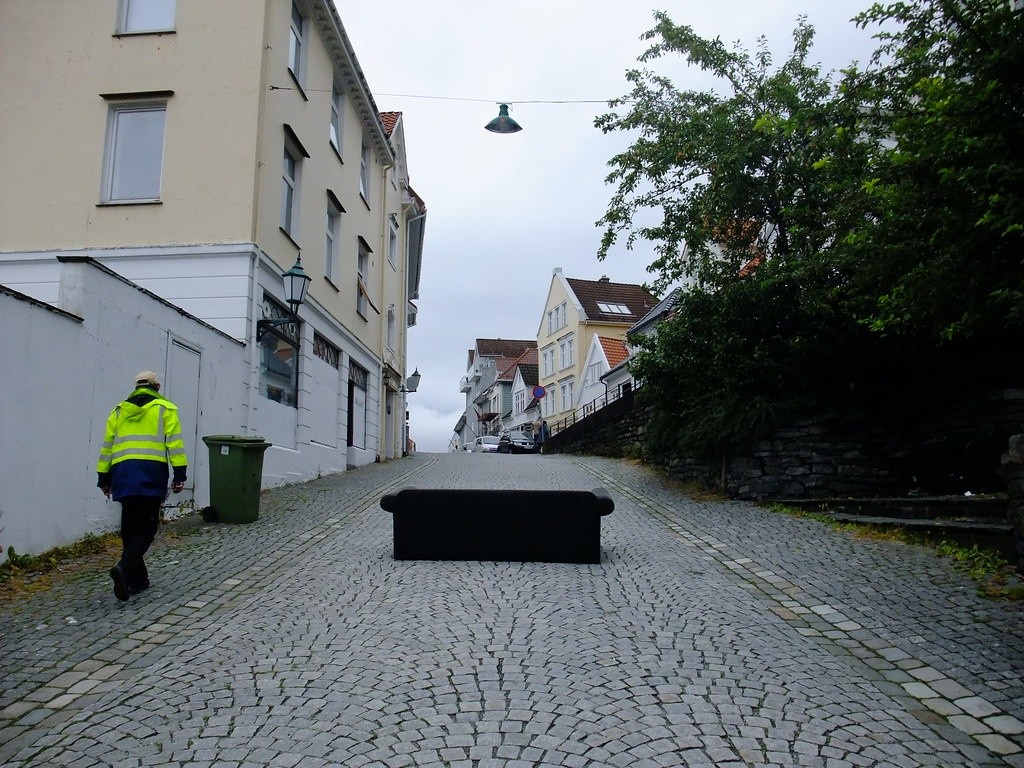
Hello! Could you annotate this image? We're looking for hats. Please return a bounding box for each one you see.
[135,370,160,387]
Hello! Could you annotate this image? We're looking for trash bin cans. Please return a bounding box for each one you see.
[202,433,272,523]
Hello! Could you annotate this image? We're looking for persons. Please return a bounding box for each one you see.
[97,370,188,601]
[534,416,550,454]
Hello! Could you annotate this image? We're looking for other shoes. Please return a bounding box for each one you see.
[110,567,130,601]
[129,579,150,594]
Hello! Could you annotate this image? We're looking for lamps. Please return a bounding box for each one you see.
[257,250,311,342]
[400,366,421,393]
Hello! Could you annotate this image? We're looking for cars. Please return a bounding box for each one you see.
[496,431,539,454]
[461,442,473,453]
[471,435,501,453]
